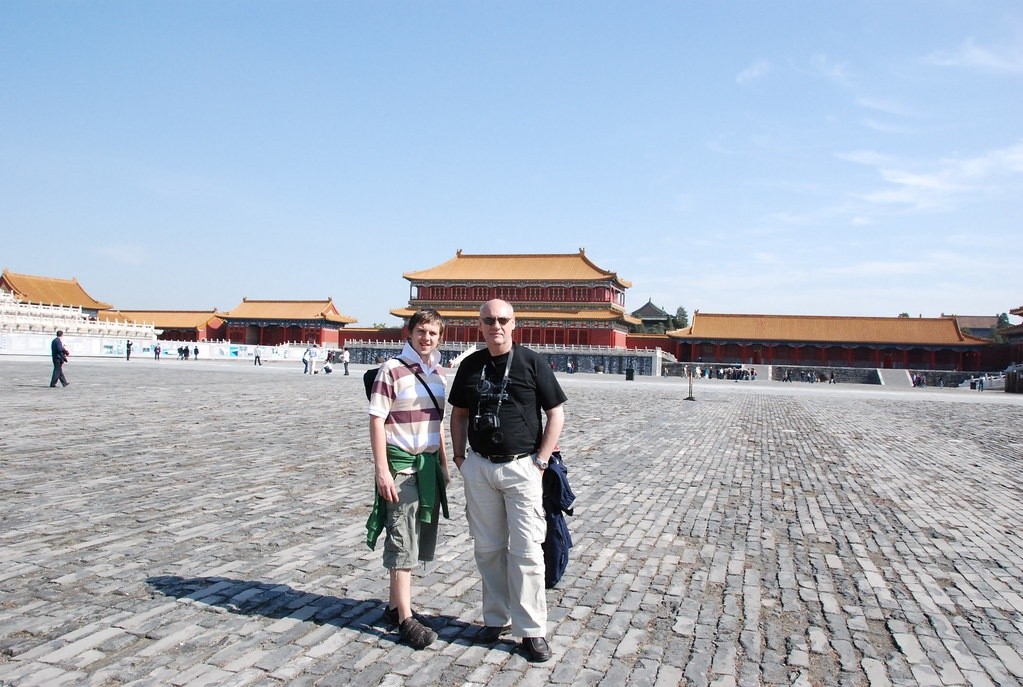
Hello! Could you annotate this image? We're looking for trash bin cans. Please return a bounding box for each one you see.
[626,368,636,380]
[970,382,976,389]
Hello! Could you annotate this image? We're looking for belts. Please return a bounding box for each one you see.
[471,449,536,464]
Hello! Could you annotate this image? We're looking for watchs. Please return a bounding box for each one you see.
[535,459,549,469]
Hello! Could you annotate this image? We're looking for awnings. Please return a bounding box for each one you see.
[320,349,333,374]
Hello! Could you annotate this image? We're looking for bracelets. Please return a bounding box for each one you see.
[453,455,465,462]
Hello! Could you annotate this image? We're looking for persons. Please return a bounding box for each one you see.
[363,307,451,650]
[340,347,350,375]
[154,343,161,361]
[978,376,986,391]
[127,340,133,360]
[683,364,758,381]
[194,346,199,360]
[50,330,70,388]
[782,369,793,383]
[913,373,927,387]
[302,344,317,375]
[177,345,190,360]
[254,344,262,366]
[801,369,817,383]
[449,298,577,661]
[566,357,576,373]
[829,370,837,385]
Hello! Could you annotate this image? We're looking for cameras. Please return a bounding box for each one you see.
[473,413,500,431]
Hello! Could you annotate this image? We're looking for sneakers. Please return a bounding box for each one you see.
[399,618,438,646]
[382,604,430,625]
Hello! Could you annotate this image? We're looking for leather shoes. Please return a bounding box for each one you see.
[475,625,512,643]
[522,635,553,661]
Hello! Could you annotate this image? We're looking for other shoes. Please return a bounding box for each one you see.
[52,386,59,388]
[64,383,70,387]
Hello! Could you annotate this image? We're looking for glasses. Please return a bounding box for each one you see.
[480,317,513,325]
